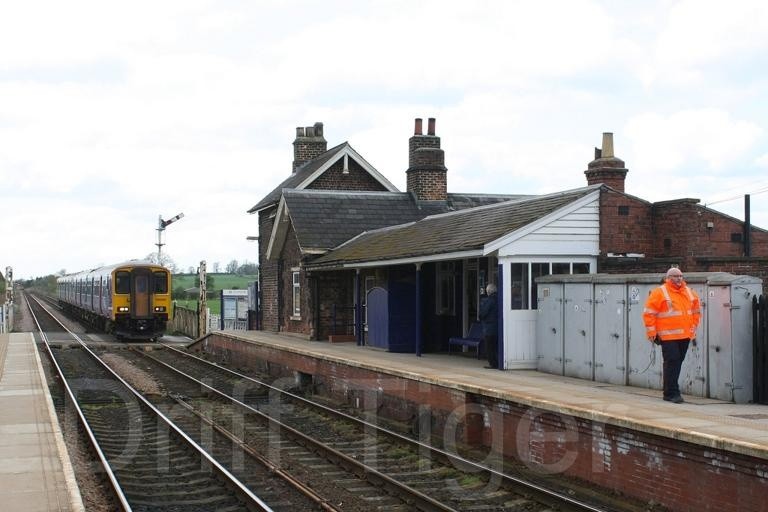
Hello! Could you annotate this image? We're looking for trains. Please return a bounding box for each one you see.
[55,260,174,342]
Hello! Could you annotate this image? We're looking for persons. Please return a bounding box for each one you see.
[643,268,701,403]
[479,284,498,369]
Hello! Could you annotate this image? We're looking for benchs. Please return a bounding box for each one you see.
[449,322,482,359]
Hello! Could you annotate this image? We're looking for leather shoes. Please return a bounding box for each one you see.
[484,361,498,369]
[662,392,684,403]
